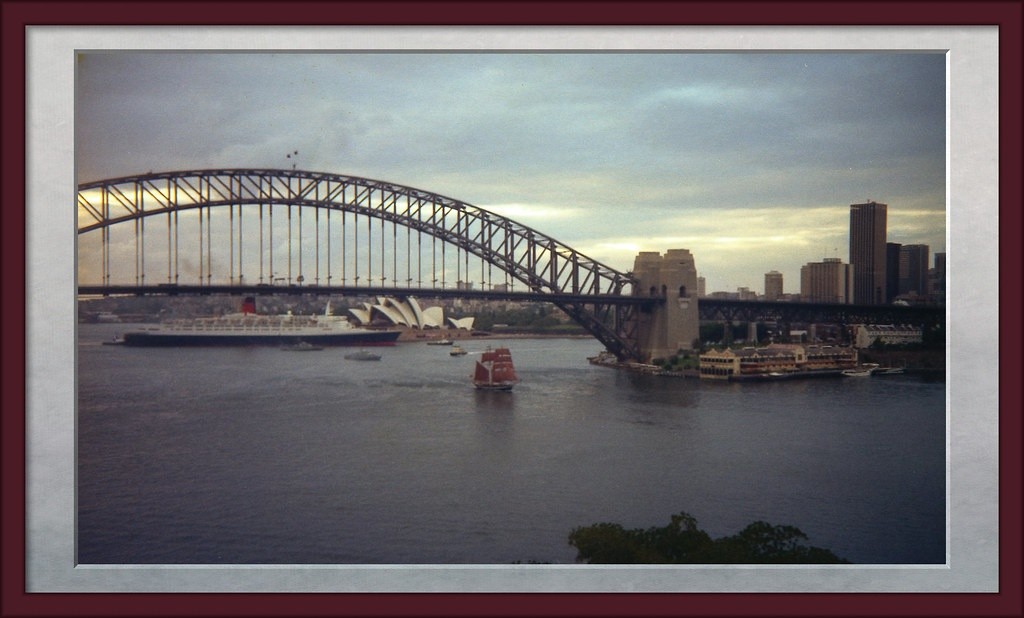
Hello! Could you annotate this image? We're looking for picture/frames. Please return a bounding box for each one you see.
[0,0,1024,618]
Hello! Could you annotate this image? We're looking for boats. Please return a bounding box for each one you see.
[472,344,517,391]
[427,337,453,345]
[449,344,468,356]
[117,309,402,347]
[284,340,323,350]
[344,348,383,361]
